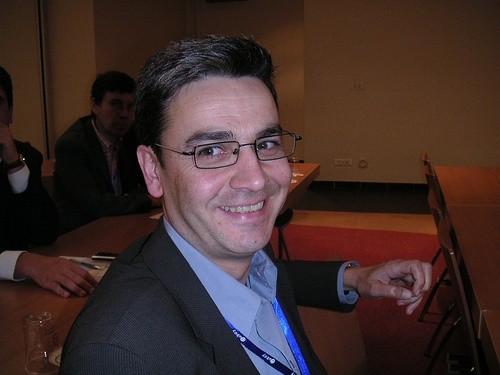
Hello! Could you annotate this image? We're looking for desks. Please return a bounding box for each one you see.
[0,160,321,375]
[432,166,500,370]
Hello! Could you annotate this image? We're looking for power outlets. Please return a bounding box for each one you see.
[334,158,352,167]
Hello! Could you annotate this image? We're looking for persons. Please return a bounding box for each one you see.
[55,70,162,233]
[57,33,433,375]
[0,66,98,298]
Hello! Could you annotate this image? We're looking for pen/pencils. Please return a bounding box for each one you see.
[69,258,99,270]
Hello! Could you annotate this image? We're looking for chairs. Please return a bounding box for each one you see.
[419,152,485,374]
[274,207,294,261]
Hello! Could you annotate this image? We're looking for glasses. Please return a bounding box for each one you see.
[155,126,302,170]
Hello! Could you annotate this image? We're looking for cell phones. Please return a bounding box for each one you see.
[91,252,119,260]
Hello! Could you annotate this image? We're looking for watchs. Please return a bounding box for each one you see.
[6,154,25,170]
